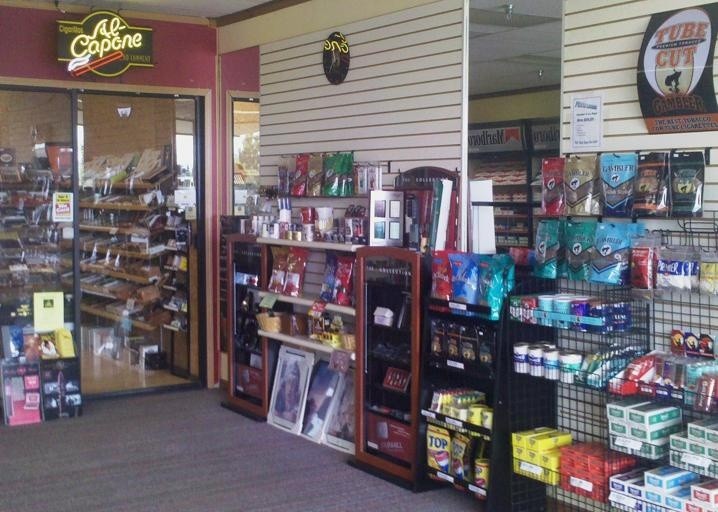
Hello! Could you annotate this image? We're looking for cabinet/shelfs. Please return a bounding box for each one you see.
[507,151,718,511]
[257,161,402,451]
[0,168,173,371]
[418,274,557,512]
[221,237,294,424]
[348,246,451,494]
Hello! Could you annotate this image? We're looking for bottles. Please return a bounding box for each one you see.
[560,355,577,384]
[270,215,280,239]
[251,215,258,238]
[570,300,589,332]
[262,215,270,237]
[537,294,552,329]
[553,297,570,329]
[474,458,489,500]
[511,342,529,374]
[544,350,560,380]
[528,344,544,377]
[469,404,488,438]
[481,408,493,441]
[259,215,264,238]
[591,298,609,336]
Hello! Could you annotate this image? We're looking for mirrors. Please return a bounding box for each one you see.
[467,0,562,259]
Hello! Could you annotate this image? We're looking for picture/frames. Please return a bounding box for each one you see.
[322,369,356,454]
[266,345,315,434]
[299,357,345,443]
